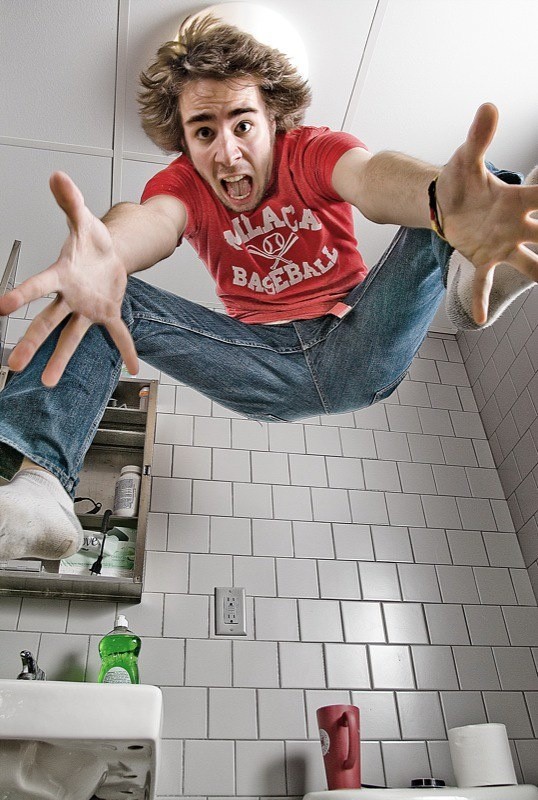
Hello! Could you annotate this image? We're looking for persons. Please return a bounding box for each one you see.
[0,11,538,561]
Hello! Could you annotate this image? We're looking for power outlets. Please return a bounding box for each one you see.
[215,587,247,635]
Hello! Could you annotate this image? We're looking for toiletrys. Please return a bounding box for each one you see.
[97,614,143,684]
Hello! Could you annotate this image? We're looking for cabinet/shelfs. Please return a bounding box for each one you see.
[0,367,158,603]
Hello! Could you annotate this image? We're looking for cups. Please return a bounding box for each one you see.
[317,704,361,791]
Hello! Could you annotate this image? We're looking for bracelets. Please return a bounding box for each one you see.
[426,176,446,243]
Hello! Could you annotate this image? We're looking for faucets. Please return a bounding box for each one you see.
[17,650,47,680]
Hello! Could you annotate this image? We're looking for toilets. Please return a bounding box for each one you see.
[303,777,537,800]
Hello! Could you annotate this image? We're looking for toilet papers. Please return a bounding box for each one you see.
[448,722,518,789]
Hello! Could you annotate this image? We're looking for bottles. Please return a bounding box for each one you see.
[113,465,142,517]
[97,614,142,684]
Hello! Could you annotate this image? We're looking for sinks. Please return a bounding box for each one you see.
[2,679,165,800]
